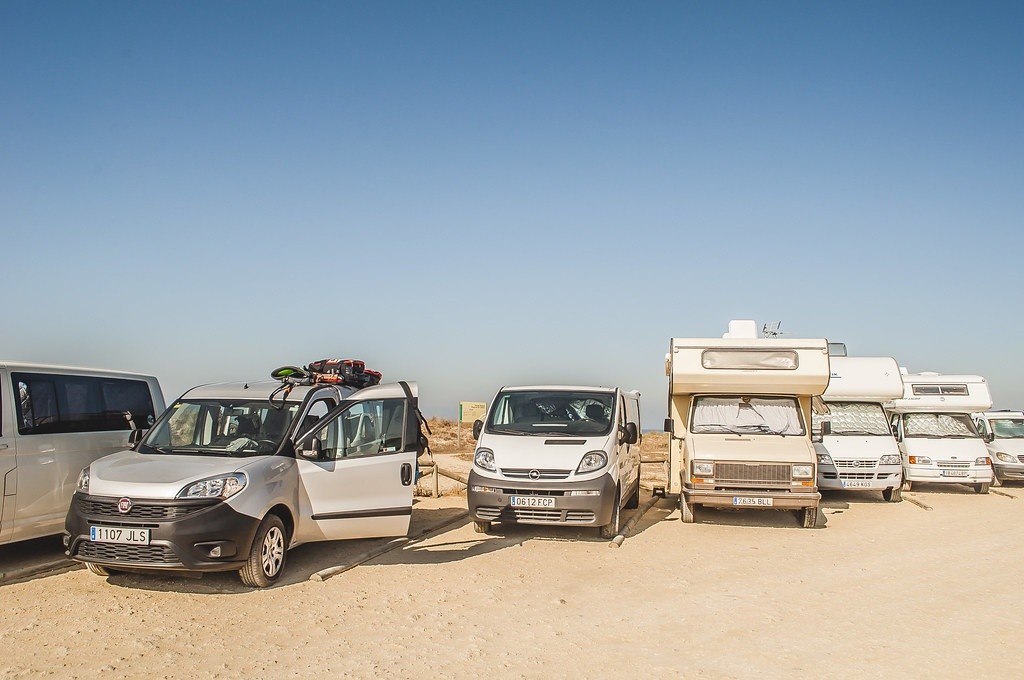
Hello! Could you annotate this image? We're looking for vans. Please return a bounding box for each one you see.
[0,361,174,546]
[467,386,642,539]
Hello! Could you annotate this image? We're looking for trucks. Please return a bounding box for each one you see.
[879,367,993,495]
[811,343,904,501]
[664,320,831,529]
[969,410,1024,488]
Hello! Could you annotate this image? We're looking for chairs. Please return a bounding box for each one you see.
[258,407,290,453]
[515,406,544,424]
[232,413,262,446]
[586,405,610,427]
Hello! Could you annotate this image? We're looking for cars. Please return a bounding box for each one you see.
[62,382,420,588]
